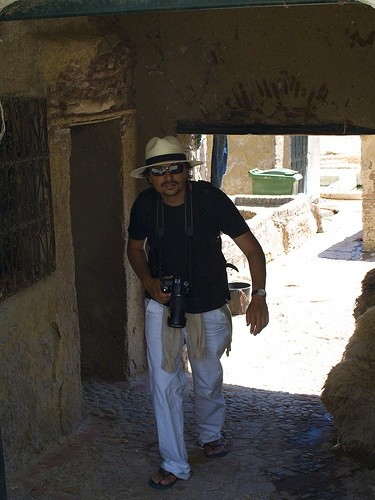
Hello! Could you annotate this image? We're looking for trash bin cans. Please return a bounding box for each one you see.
[248,167,303,195]
[227,282,251,316]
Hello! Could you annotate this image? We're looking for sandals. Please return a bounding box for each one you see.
[148,469,177,489]
[202,442,228,457]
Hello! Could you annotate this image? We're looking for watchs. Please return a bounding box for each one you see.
[252,288,266,297]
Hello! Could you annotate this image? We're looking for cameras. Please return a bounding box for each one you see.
[160,277,191,329]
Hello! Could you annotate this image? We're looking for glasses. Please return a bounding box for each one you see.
[150,164,186,176]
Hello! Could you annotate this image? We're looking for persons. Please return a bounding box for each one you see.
[125,135,270,490]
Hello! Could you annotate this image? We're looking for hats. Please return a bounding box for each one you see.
[130,135,203,179]
